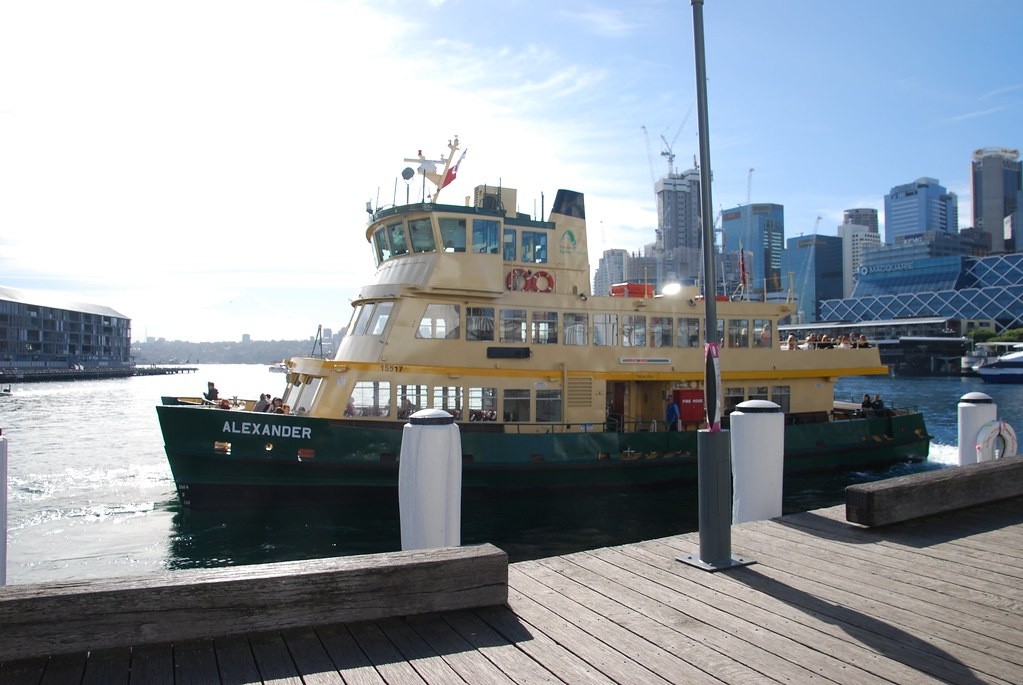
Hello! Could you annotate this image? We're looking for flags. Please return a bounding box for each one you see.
[424,165,457,188]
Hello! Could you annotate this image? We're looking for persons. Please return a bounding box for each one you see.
[665,394,679,430]
[761,323,771,343]
[218,398,230,410]
[298,406,305,413]
[204,382,218,405]
[547,323,557,344]
[860,393,883,409]
[741,329,749,342]
[252,393,290,414]
[787,332,869,349]
[343,393,494,420]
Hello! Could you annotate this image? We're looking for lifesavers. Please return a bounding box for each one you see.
[975,419,1018,463]
[531,271,554,292]
[507,268,531,291]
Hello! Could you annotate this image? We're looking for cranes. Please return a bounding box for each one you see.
[659,99,698,175]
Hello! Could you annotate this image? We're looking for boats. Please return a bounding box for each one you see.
[961,341,1023,384]
[154,134,936,516]
